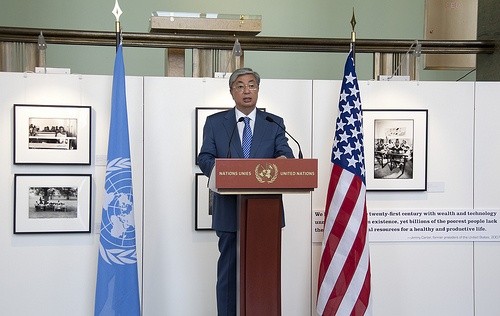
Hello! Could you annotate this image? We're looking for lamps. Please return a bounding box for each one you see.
[222,37,243,78]
[36,32,48,73]
[388,38,423,80]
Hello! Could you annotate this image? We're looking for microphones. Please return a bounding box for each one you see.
[227,117,245,158]
[266,117,304,159]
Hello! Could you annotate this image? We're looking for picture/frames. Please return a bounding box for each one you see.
[195,173,214,231]
[13,174,93,235]
[360,107,430,193]
[12,104,92,166]
[195,107,266,167]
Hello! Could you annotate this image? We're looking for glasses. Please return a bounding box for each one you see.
[230,82,259,91]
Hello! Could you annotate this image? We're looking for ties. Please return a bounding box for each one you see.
[242,117,253,159]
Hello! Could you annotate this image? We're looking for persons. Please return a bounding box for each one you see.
[377,138,412,168]
[29,124,68,144]
[198,67,295,316]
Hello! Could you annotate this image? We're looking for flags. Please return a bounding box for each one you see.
[94,38,140,316]
[317,47,371,316]
